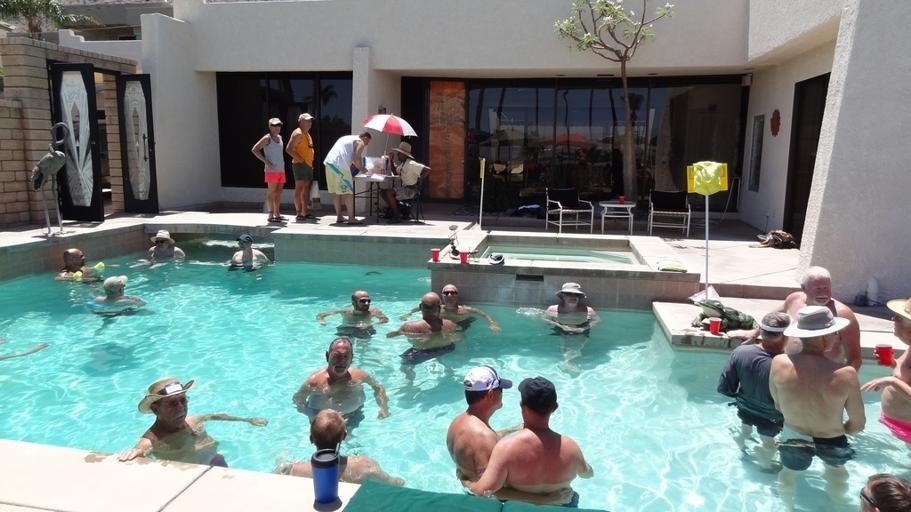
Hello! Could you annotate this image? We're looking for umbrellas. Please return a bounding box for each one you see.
[362,112,419,157]
[537,133,574,163]
[479,128,533,164]
[559,133,601,163]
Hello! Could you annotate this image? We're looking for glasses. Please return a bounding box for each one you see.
[360,299,370,303]
[168,395,188,406]
[443,291,455,295]
[155,240,169,244]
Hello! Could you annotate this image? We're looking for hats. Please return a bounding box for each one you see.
[758,311,793,332]
[103,275,128,292]
[518,376,557,407]
[555,282,585,300]
[783,305,850,338]
[268,118,283,126]
[236,234,253,243]
[138,376,194,413]
[299,113,314,120]
[464,365,512,392]
[886,297,911,320]
[150,230,175,245]
[392,142,415,159]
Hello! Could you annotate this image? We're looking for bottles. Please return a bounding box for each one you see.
[866,276,880,306]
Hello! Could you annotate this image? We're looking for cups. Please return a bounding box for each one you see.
[461,252,468,263]
[874,344,894,366]
[620,196,625,204]
[431,248,441,262]
[709,317,723,336]
[856,295,866,306]
[310,449,343,503]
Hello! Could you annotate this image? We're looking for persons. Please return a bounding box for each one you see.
[446,363,574,506]
[387,292,461,392]
[270,409,407,489]
[859,472,911,512]
[54,247,105,285]
[285,113,322,222]
[719,312,795,476]
[292,337,391,432]
[117,375,269,463]
[316,288,389,342]
[324,130,372,226]
[252,116,290,224]
[859,294,911,451]
[542,282,601,376]
[93,274,147,310]
[741,265,864,375]
[399,283,504,335]
[454,375,593,506]
[224,234,274,268]
[380,142,433,224]
[147,229,186,263]
[769,305,866,506]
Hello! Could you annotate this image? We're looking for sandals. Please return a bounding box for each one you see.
[268,216,288,222]
[296,214,315,220]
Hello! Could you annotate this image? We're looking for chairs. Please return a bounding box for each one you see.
[386,177,424,223]
[646,188,692,238]
[543,186,594,235]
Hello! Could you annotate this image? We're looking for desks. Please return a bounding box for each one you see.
[348,175,392,222]
[597,198,636,233]
